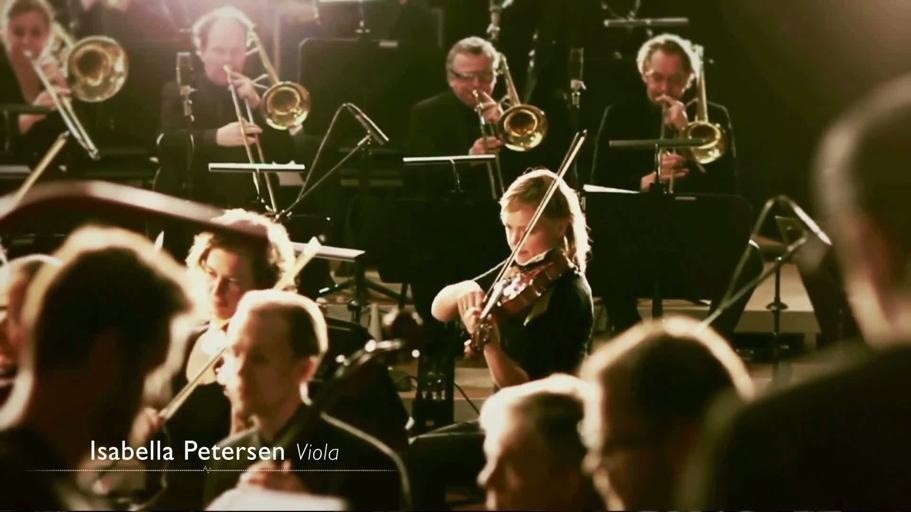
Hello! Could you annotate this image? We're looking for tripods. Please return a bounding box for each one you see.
[318,190,414,305]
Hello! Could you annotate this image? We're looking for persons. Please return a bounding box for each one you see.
[1,1,909,509]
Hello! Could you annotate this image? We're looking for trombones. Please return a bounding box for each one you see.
[26,22,130,163]
[655,44,729,196]
[223,27,312,215]
[474,54,548,202]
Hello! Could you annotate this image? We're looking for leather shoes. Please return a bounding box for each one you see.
[728,335,756,362]
[614,310,643,334]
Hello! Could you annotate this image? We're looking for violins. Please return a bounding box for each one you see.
[185,329,228,385]
[468,256,565,350]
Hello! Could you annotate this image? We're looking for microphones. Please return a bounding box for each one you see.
[569,48,585,89]
[778,194,831,246]
[339,101,392,149]
[175,51,194,88]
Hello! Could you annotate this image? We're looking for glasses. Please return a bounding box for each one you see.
[448,68,494,83]
